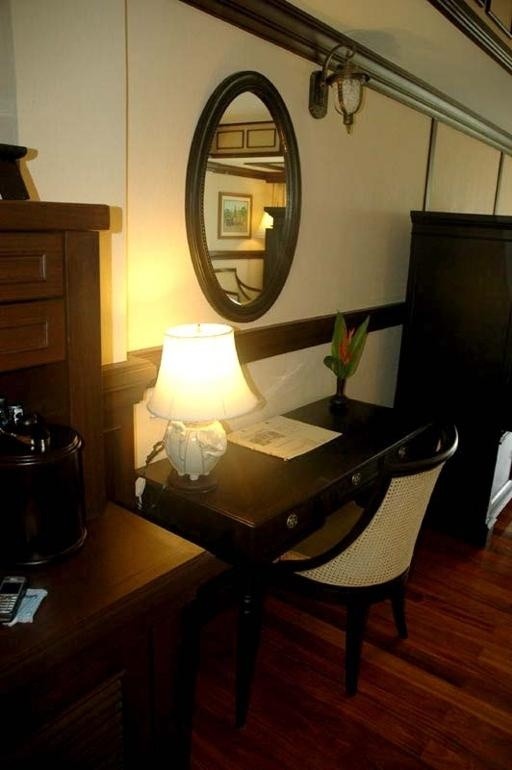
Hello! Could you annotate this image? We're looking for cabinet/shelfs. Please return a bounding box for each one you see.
[0,198,231,770]
[392,209,511,551]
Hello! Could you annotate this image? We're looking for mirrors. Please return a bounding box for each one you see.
[184,68,303,323]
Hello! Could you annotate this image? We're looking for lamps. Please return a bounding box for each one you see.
[145,321,259,498]
[307,40,371,135]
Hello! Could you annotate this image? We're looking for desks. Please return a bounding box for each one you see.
[134,394,442,729]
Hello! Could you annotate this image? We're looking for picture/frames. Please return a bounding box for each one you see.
[217,190,253,239]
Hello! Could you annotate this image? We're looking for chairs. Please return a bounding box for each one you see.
[248,418,464,698]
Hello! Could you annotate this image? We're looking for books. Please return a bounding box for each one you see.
[226,415,343,462]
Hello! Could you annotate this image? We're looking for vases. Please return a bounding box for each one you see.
[327,379,347,413]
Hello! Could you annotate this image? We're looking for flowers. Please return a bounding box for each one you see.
[324,310,372,378]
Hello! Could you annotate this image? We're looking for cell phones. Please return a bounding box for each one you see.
[0,575,27,622]
